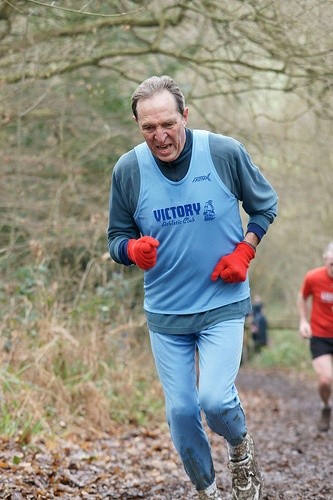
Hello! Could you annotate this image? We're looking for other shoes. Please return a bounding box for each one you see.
[228,436,265,500]
[318,407,331,432]
[198,481,230,500]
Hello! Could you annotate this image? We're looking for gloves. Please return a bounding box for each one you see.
[211,241,255,284]
[127,235,160,270]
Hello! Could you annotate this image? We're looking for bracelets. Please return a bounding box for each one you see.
[241,240,256,253]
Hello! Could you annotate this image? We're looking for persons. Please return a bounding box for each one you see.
[107,74,279,500]
[299,245,333,438]
[249,294,269,356]
